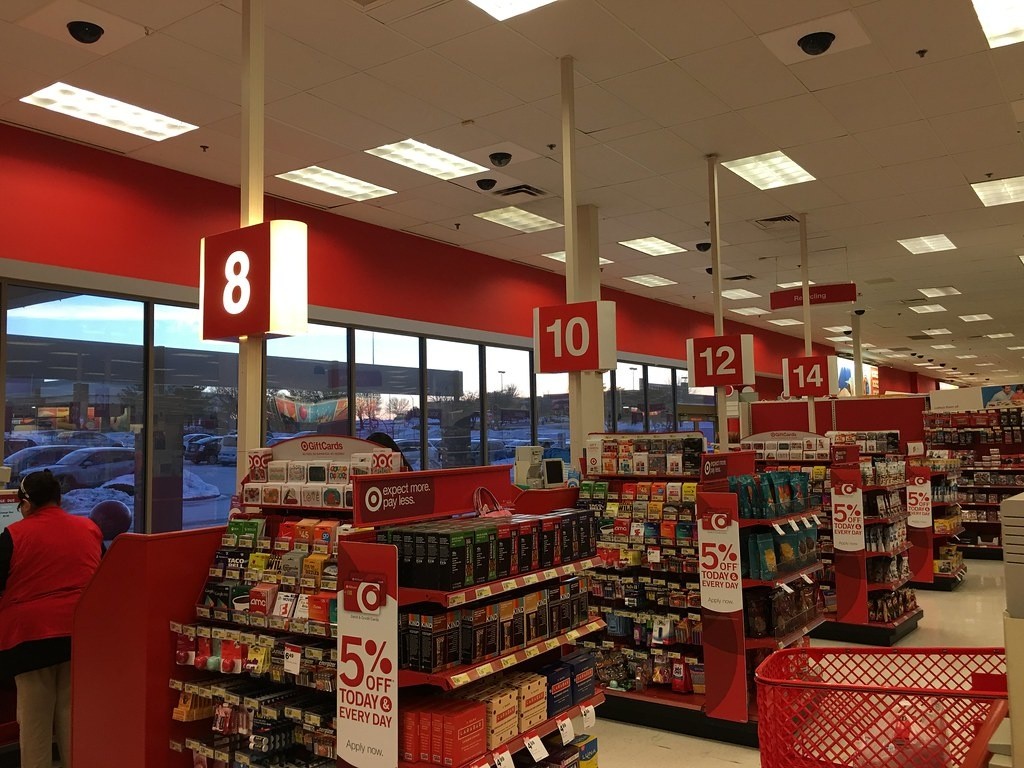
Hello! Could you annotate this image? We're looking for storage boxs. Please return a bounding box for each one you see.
[374,508,599,767]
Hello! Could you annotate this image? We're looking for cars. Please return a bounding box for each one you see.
[395,439,437,468]
[0,429,136,494]
[184,431,237,465]
[471,433,570,461]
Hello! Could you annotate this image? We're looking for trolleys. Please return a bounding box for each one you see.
[754,645,1010,768]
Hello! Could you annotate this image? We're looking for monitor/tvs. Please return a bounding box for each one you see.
[543,458,564,488]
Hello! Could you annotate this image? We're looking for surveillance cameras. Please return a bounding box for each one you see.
[706,268,712,275]
[477,179,497,191]
[797,33,835,55]
[985,379,989,380]
[489,153,512,167]
[951,380,953,382]
[843,331,852,335]
[911,353,975,375]
[67,21,104,44]
[855,310,865,315]
[696,243,711,252]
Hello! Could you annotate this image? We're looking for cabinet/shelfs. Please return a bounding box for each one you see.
[696,451,822,751]
[168,436,408,768]
[828,445,923,647]
[903,451,966,591]
[921,406,1024,560]
[579,431,706,737]
[338,464,607,768]
[740,431,836,641]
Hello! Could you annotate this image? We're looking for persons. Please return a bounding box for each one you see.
[551,432,569,449]
[0,468,108,768]
[990,385,1024,400]
